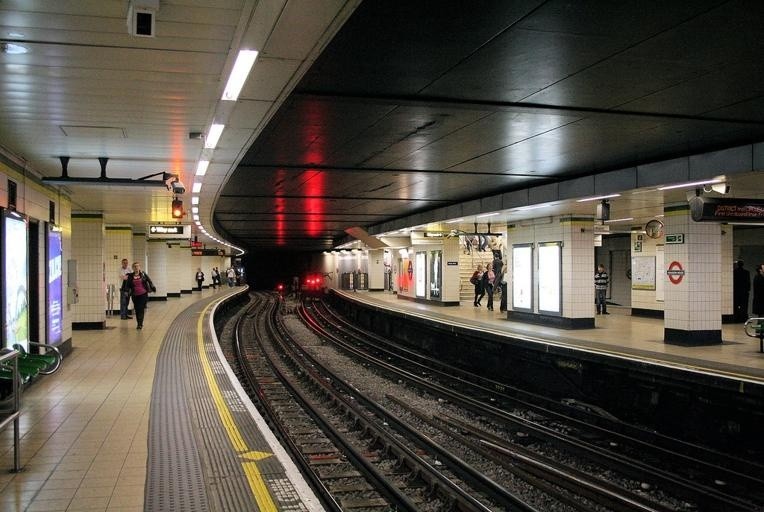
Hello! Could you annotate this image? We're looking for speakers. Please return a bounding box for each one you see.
[596,203,611,220]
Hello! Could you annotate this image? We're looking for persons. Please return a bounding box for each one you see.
[195,264,241,291]
[594,264,610,314]
[733,259,764,333]
[466,236,507,313]
[117,259,133,319]
[124,262,156,329]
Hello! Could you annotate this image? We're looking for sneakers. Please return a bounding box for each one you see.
[121,315,133,320]
[467,247,486,255]
[473,302,481,307]
[487,304,494,311]
[136,324,143,330]
[597,311,610,315]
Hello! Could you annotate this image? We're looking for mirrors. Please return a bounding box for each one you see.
[645,219,664,239]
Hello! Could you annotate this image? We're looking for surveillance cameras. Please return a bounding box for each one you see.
[170,180,186,194]
[447,234,455,239]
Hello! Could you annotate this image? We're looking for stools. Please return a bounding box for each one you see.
[0,342,62,413]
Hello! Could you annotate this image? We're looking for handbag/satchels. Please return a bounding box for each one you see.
[142,271,152,292]
[470,277,478,284]
